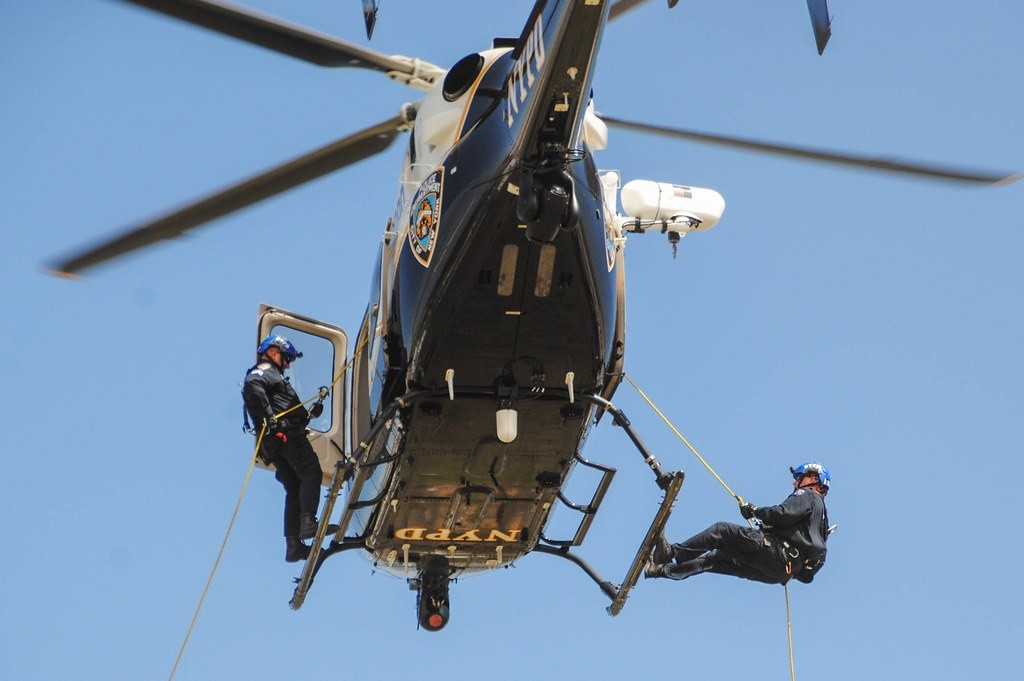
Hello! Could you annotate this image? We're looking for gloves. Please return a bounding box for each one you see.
[740,502,757,520]
[268,416,284,434]
[308,404,323,419]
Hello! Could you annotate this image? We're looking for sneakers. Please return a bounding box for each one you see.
[285,537,324,563]
[653,528,674,564]
[299,512,340,538]
[644,551,664,578]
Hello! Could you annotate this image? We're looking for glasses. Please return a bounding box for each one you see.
[283,353,292,364]
[796,475,802,481]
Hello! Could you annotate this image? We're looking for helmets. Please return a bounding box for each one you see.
[790,463,830,491]
[257,335,302,362]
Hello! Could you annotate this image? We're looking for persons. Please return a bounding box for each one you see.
[644,463,830,584]
[242,335,341,564]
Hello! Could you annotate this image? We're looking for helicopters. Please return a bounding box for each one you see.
[42,0,1021,617]
